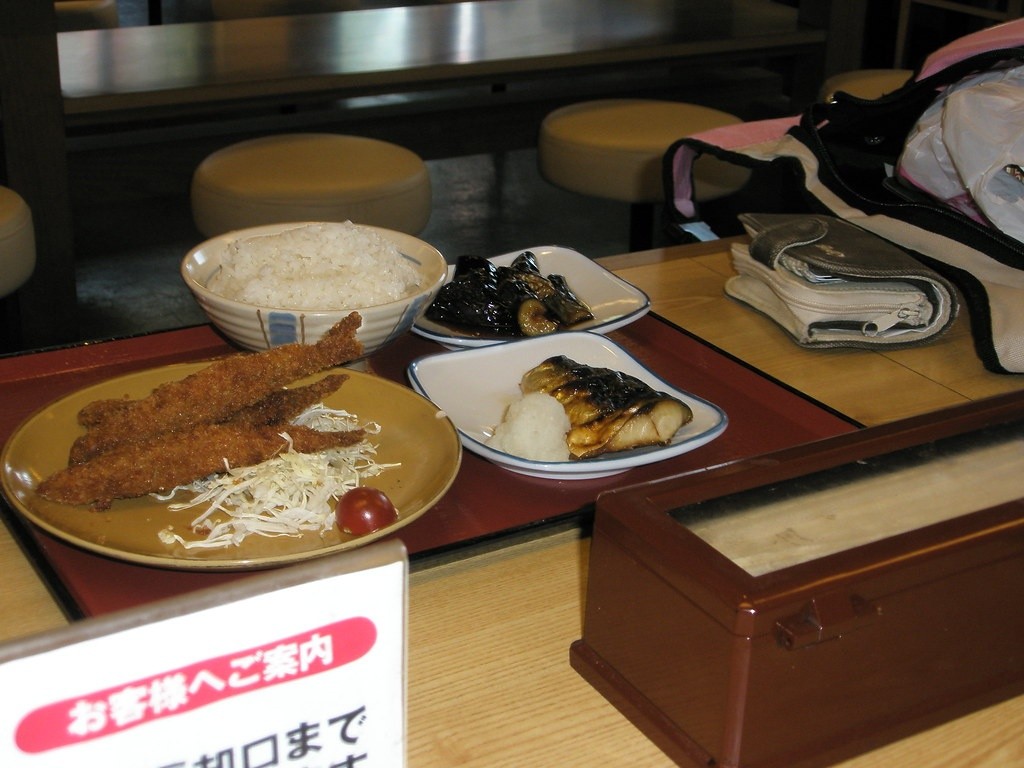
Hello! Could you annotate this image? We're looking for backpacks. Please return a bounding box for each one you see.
[660,18,1024,374]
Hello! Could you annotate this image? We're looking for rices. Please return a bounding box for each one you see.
[208,219,424,312]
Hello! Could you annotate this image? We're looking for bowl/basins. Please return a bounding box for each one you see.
[181,221,447,358]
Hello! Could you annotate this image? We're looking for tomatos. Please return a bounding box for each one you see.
[333,486,398,535]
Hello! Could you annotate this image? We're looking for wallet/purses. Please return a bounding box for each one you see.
[725,217,961,351]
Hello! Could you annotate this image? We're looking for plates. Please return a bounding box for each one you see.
[2,360,462,570]
[406,332,730,480]
[412,244,653,351]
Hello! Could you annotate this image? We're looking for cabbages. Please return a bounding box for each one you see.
[149,402,404,552]
